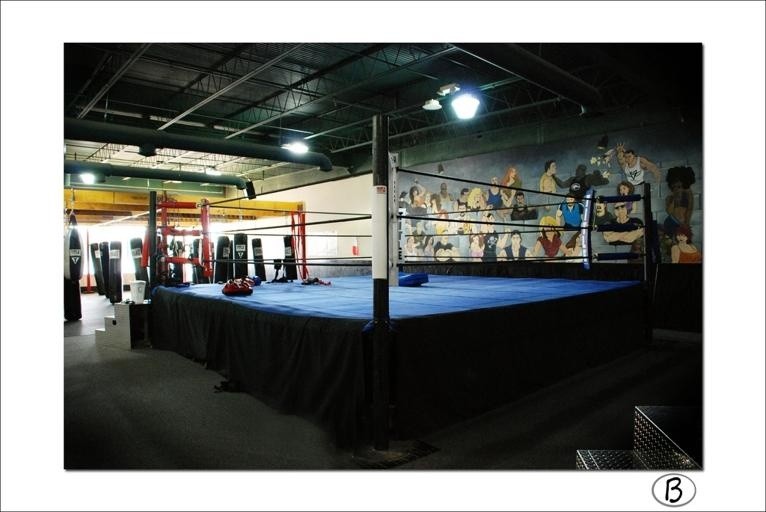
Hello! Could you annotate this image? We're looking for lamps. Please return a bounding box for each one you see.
[422,98,443,111]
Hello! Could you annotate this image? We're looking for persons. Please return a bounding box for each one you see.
[396,138,703,263]
